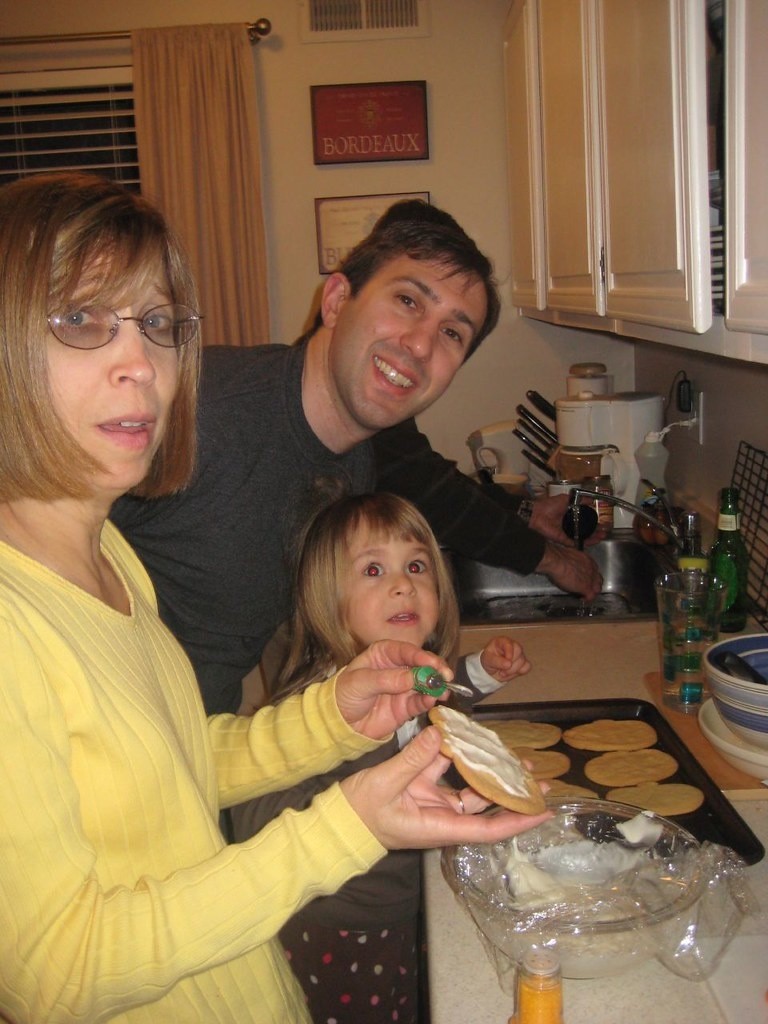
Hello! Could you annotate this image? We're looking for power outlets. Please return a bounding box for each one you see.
[687,391,703,446]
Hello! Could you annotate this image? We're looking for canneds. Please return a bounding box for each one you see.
[582,475,614,533]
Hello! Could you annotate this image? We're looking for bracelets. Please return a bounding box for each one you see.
[519,499,534,524]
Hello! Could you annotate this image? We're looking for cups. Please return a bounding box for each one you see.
[654,569,727,713]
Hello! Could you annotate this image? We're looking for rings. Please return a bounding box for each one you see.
[450,790,465,815]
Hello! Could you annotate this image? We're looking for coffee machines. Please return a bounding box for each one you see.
[554,390,666,527]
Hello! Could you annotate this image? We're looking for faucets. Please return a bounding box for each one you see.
[568,475,703,559]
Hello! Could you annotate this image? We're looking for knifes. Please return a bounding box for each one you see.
[511,390,557,477]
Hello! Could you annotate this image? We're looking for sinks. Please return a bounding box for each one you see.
[448,538,678,626]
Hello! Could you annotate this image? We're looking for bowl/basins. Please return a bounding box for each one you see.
[700,632,768,749]
[439,797,711,982]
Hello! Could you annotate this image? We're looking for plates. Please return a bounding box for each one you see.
[709,168,725,313]
[697,698,768,780]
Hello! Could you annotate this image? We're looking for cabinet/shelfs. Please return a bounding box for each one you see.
[502,0,768,370]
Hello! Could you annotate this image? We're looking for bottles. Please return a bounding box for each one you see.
[512,951,563,1024]
[709,488,747,633]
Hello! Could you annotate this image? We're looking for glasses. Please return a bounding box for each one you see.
[44,303,205,349]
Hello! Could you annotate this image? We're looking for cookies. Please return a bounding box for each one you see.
[427,704,546,814]
[481,718,705,816]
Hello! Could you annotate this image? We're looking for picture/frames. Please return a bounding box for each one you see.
[311,80,429,164]
[315,191,431,274]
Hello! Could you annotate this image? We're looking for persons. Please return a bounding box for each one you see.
[0,175,602,1024]
